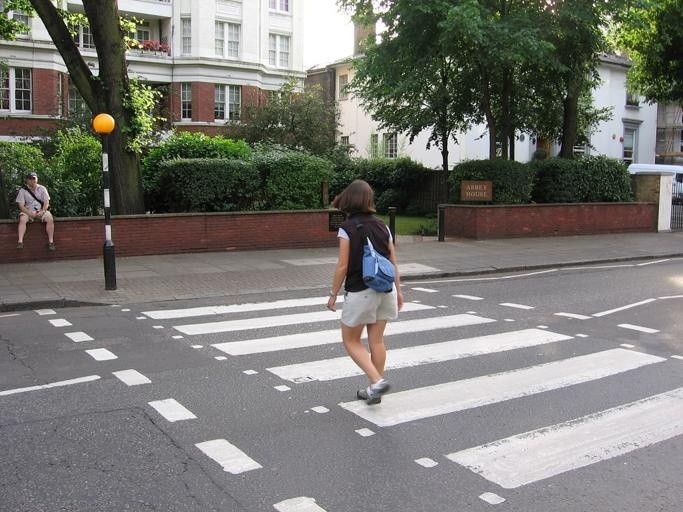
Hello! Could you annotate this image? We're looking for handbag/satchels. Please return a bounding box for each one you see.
[361,234,396,293]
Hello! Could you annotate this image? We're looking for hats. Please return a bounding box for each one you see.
[27,172,37,178]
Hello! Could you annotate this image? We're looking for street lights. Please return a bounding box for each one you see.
[93,113,118,292]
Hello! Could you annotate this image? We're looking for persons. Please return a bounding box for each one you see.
[327,179,403,405]
[15,172,56,252]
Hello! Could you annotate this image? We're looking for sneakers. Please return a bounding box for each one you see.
[358,378,391,406]
[16,241,23,249]
[48,242,56,251]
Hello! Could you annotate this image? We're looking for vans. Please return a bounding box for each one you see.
[625,163,682,206]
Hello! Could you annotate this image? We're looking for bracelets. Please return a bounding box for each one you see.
[43,211,45,214]
[330,290,337,297]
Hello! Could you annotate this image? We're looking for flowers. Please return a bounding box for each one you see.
[128,39,170,52]
